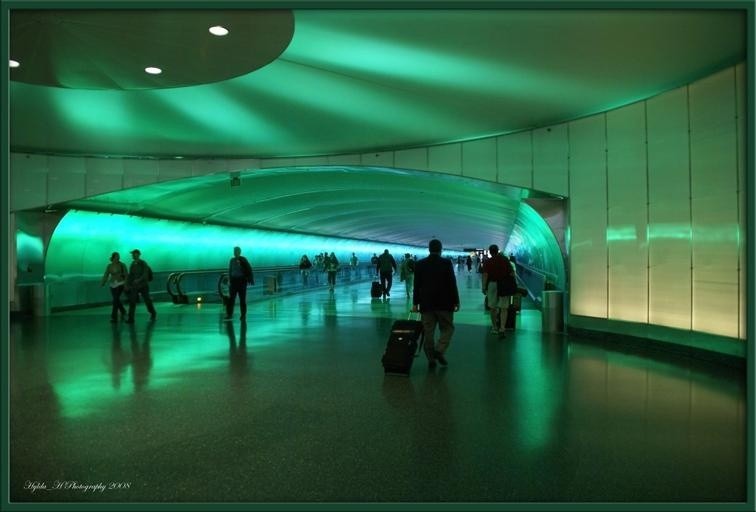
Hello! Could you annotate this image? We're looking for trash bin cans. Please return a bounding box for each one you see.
[541,290,564,333]
[28,283,44,316]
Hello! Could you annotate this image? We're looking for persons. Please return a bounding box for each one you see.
[482,245,515,339]
[413,240,460,369]
[125,249,156,324]
[100,253,128,322]
[224,246,255,320]
[299,250,516,298]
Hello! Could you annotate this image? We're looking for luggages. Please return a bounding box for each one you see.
[499,296,516,332]
[371,276,382,298]
[381,310,424,376]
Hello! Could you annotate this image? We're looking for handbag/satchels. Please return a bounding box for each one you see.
[498,275,516,296]
[127,286,139,302]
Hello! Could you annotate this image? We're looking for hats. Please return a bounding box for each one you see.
[131,250,140,254]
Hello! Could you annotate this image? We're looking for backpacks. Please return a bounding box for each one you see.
[142,261,153,282]
